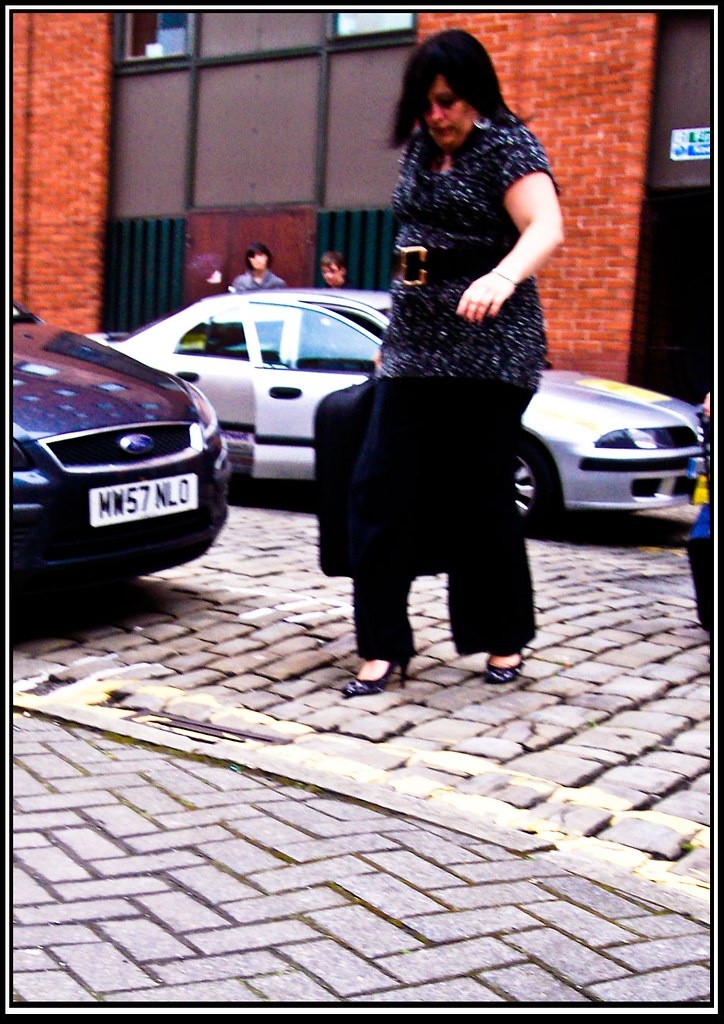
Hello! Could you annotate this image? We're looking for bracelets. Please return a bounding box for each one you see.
[492,269,518,288]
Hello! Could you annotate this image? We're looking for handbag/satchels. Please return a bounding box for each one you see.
[312,378,375,578]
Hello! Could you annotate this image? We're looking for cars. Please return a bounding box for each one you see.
[85,288,704,542]
[13,299,233,645]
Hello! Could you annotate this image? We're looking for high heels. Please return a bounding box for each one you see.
[339,655,411,697]
[484,651,523,683]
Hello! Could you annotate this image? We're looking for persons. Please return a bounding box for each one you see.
[231,242,288,292]
[321,251,349,289]
[339,29,564,696]
